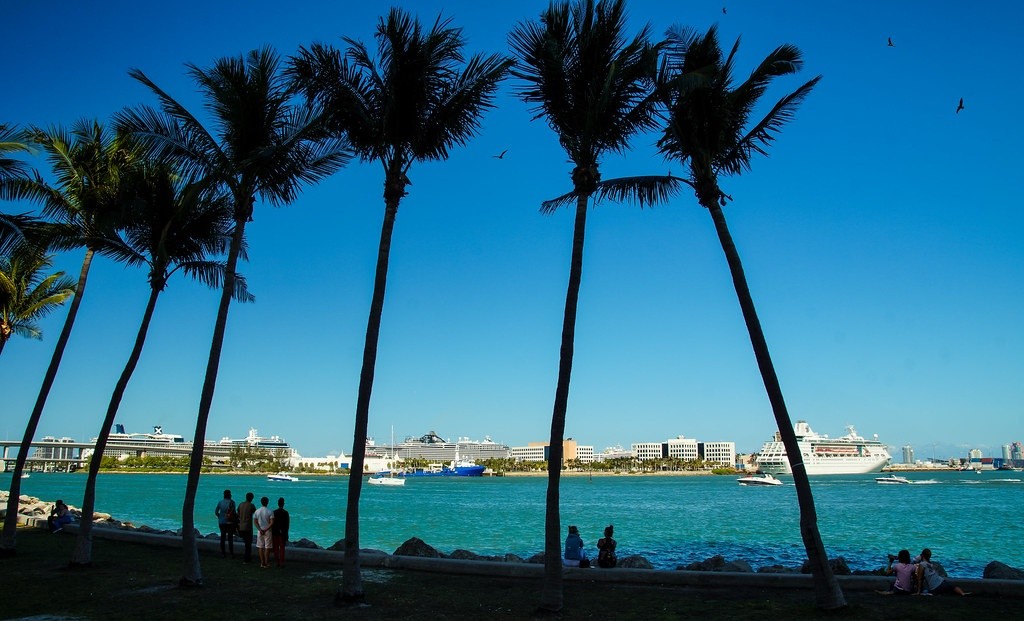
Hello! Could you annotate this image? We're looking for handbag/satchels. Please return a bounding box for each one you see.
[579,559,590,567]
[226,501,236,522]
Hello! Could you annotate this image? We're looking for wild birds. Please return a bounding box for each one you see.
[492,150,508,158]
[956,97,964,114]
[885,37,893,46]
[721,6,727,15]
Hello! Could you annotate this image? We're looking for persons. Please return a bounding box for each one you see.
[254,497,274,568]
[273,497,289,571]
[48,500,67,532]
[564,526,586,560]
[51,504,75,535]
[912,548,972,596]
[236,493,257,563]
[597,524,617,568]
[215,489,238,561]
[885,550,916,594]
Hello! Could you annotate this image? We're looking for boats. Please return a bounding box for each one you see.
[268,472,299,482]
[374,466,488,477]
[882,460,975,472]
[368,476,405,487]
[875,476,910,486]
[21,473,31,479]
[737,474,783,486]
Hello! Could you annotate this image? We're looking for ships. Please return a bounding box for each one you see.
[756,421,893,475]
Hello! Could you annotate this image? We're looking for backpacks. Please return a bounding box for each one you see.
[602,550,617,568]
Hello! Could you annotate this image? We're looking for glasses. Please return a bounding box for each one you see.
[920,554,922,555]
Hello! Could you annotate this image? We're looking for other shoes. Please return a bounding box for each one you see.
[280,564,285,568]
[242,561,249,565]
[261,564,272,569]
[276,563,280,566]
[53,528,64,535]
[48,531,53,535]
[962,591,975,598]
